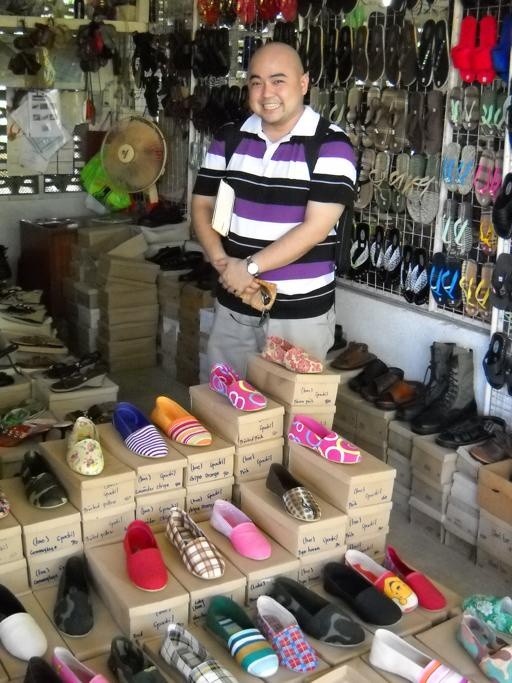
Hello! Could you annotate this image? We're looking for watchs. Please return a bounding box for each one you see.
[246,256,262,277]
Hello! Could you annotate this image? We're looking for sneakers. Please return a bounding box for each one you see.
[290,414,362,463]
[1,285,110,519]
[211,498,272,561]
[167,507,226,579]
[261,335,323,374]
[210,363,267,412]
[113,400,169,459]
[266,464,321,522]
[151,395,212,447]
[122,520,168,592]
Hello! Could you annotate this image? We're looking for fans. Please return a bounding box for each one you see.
[100,115,168,206]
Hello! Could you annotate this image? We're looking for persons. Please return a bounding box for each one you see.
[191,40,360,384]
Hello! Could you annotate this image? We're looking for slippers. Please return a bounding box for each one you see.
[259,1,346,112]
[330,323,512,465]
[133,0,256,134]
[339,0,512,321]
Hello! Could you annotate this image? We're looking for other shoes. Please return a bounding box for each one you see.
[1,585,48,661]
[458,615,511,682]
[53,646,105,682]
[25,655,55,681]
[105,635,166,682]
[160,548,463,682]
[54,556,94,635]
